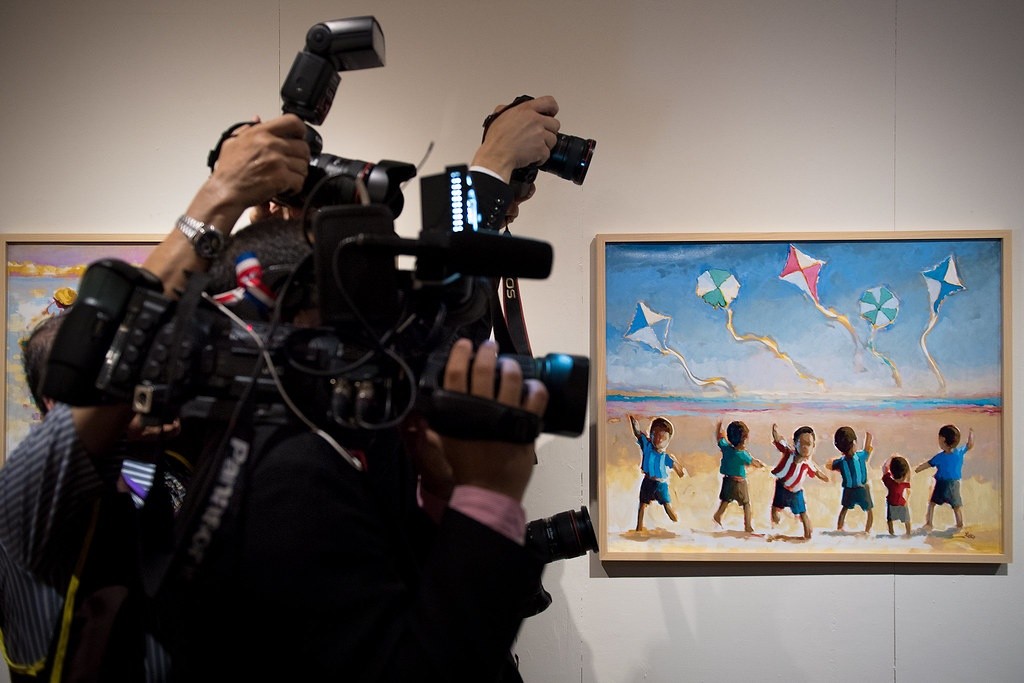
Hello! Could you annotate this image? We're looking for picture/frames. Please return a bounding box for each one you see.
[1,234,167,467]
[594,231,1014,563]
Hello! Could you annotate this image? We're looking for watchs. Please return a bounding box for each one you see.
[176,212,230,263]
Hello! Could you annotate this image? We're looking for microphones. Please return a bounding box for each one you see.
[359,230,553,277]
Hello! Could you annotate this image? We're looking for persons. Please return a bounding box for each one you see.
[1,93,560,683]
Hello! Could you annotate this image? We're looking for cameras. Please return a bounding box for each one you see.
[482,96,597,202]
[519,505,599,617]
[268,16,416,221]
